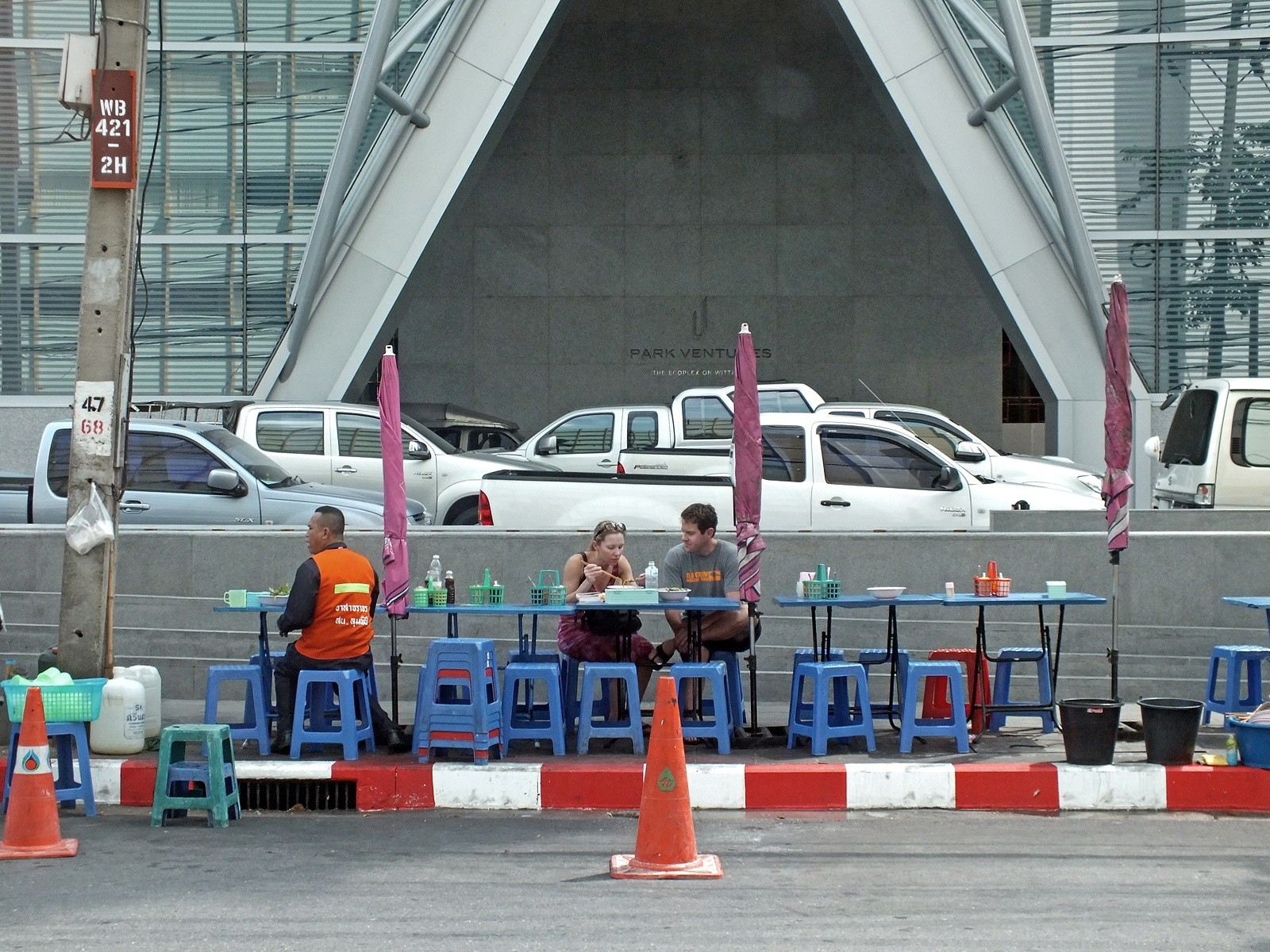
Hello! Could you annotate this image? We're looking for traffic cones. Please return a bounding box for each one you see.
[0,687,78,862]
[606,674,725,879]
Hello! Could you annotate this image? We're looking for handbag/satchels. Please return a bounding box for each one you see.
[65,482,116,556]
[584,609,642,636]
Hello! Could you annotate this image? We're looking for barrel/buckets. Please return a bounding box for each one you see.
[90,663,162,755]
[1059,696,1206,764]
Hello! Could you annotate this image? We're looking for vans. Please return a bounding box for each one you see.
[1150,378,1270,512]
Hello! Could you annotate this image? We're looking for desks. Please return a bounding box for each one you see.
[1221,594,1270,645]
[771,591,944,746]
[211,589,743,733]
[932,589,1110,746]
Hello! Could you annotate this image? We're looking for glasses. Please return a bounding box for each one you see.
[594,522,627,538]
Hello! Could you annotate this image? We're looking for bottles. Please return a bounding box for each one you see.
[423,554,455,604]
[645,561,659,589]
[1225,733,1239,767]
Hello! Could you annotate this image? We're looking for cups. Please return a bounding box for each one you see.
[224,589,247,607]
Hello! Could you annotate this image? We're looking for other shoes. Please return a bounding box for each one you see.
[271,730,292,753]
[386,730,411,752]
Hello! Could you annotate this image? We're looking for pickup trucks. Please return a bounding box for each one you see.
[478,412,1105,532]
[0,417,431,530]
[233,402,558,527]
[617,402,1107,512]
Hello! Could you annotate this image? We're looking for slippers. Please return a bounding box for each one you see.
[642,724,652,738]
[646,642,674,671]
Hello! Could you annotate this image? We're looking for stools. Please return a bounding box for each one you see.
[1203,644,1269,735]
[202,642,1058,761]
[1,722,96,818]
[149,723,245,830]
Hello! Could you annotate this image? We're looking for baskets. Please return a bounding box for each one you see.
[469,568,504,605]
[802,563,842,600]
[972,560,1012,598]
[530,569,567,606]
[1,666,107,722]
[413,574,448,608]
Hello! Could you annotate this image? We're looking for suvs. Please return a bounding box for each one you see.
[461,379,829,485]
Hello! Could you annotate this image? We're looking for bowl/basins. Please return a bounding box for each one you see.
[865,586,906,598]
[657,587,691,602]
[257,594,290,606]
[1228,710,1270,771]
[606,585,644,589]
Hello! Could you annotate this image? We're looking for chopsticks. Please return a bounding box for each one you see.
[1225,712,1251,722]
[581,561,621,581]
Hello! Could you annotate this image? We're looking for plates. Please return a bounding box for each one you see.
[1250,701,1270,726]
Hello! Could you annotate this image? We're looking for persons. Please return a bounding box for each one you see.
[557,523,655,739]
[646,503,764,746]
[267,505,407,752]
